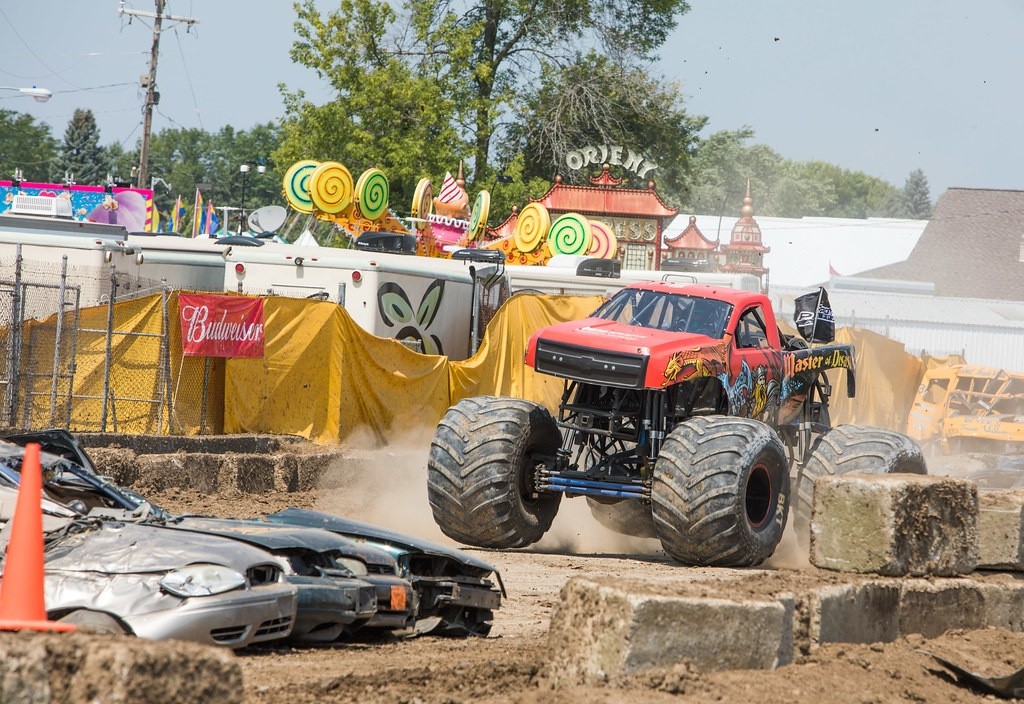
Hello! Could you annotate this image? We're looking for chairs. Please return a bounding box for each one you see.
[678,301,743,348]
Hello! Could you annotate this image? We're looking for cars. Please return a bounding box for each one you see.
[0,424,509,652]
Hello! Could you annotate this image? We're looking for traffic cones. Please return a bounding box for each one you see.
[0,441,79,634]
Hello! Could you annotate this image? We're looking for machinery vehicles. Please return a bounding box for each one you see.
[423,273,927,570]
